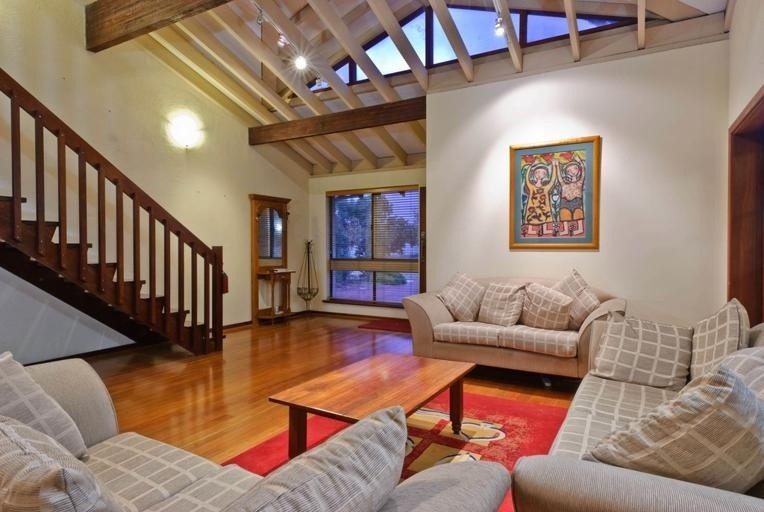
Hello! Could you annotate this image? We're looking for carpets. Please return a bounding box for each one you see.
[221,387,568,512]
[358,319,411,333]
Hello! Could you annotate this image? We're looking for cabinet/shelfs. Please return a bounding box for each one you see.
[252,269,296,326]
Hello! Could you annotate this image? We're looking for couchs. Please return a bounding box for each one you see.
[403,274,626,379]
[510,320,764,512]
[0,357,510,512]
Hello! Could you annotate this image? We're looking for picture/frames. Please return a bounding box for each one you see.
[509,135,600,249]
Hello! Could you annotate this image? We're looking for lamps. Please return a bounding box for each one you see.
[277,35,290,48]
[495,18,505,34]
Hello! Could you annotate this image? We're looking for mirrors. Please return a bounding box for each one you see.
[253,201,287,269]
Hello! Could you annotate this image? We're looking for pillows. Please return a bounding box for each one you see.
[590,298,764,493]
[436,269,600,330]
